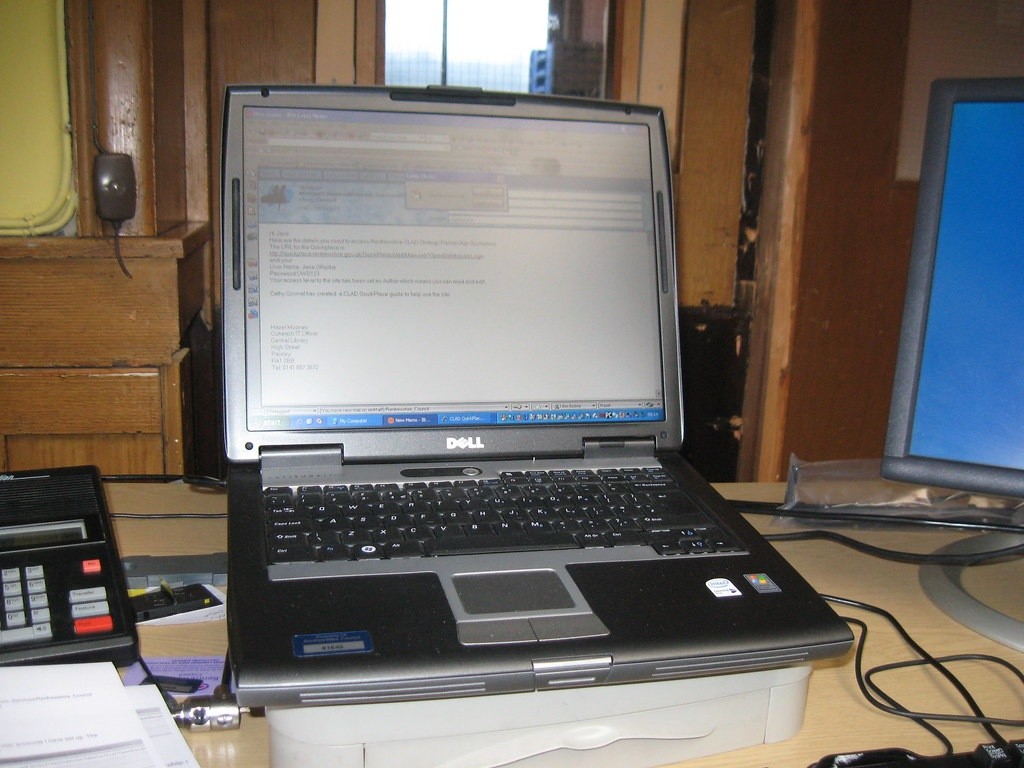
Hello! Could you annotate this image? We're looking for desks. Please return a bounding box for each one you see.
[3,477,1024,768]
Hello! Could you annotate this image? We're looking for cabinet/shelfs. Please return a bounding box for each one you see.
[0,221,217,483]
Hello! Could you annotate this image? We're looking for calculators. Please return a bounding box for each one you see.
[0,465,141,670]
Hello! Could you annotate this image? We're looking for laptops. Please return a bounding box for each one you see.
[219,85,853,710]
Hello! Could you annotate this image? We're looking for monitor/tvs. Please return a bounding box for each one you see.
[880,76,1024,653]
